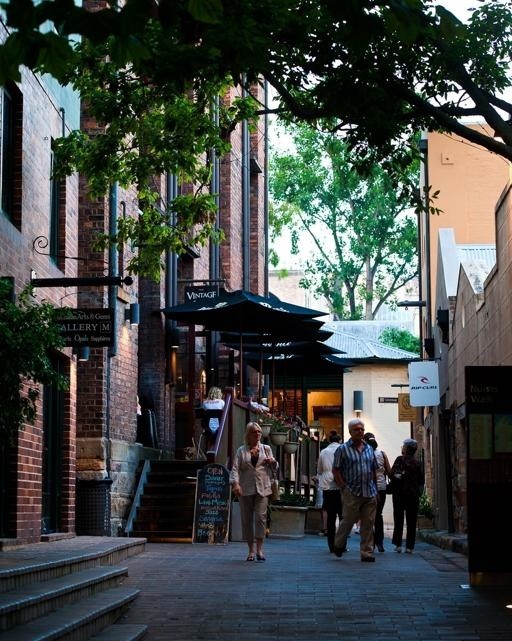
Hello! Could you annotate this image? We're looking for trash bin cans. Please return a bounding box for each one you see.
[75,479,112,535]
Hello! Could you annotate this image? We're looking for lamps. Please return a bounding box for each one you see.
[125,303,180,349]
[354,390,363,419]
[73,347,91,363]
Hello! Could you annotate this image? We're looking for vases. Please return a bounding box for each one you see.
[259,424,300,453]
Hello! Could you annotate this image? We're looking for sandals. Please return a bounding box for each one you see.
[257,554,265,560]
[247,551,255,561]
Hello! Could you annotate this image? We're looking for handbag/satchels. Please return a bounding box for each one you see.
[271,478,280,502]
[386,481,393,494]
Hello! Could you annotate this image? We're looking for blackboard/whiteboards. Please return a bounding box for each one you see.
[193,463,232,544]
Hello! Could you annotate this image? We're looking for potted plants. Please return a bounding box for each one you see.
[269,493,310,539]
[416,490,434,529]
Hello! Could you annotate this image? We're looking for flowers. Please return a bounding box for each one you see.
[253,407,310,444]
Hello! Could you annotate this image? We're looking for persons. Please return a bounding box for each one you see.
[330,419,379,564]
[310,458,327,537]
[316,430,350,554]
[363,433,392,553]
[386,438,425,555]
[227,421,280,563]
[202,387,225,451]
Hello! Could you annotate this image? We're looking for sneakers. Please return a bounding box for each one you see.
[334,546,342,557]
[378,548,412,553]
[361,556,375,562]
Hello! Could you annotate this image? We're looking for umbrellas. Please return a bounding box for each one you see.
[154,287,361,405]
